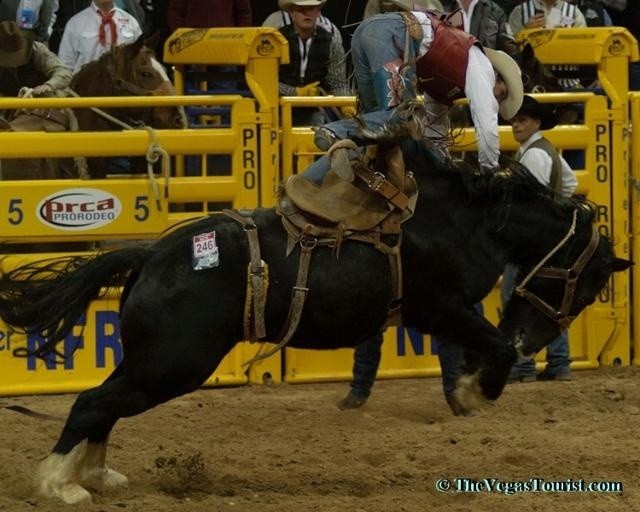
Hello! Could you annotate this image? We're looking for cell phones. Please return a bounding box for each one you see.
[535,9,544,18]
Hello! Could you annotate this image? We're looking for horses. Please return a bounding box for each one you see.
[0,31,188,255]
[0,132,637,506]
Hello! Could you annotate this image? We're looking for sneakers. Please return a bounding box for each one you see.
[313,126,338,151]
[508,368,572,383]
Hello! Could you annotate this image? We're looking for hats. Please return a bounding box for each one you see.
[511,95,558,131]
[482,46,525,121]
[281,0,324,11]
[0,20,37,70]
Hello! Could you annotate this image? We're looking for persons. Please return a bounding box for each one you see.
[259,0,615,129]
[48,1,155,57]
[0,20,72,124]
[338,329,466,409]
[1,1,60,52]
[58,0,143,81]
[499,95,579,381]
[279,12,527,190]
